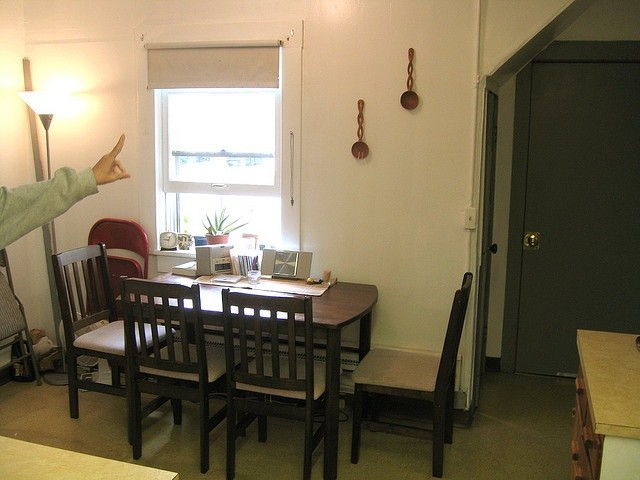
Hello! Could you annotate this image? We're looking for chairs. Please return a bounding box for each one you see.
[118,274,247,473]
[350,271,473,478]
[220,285,325,479]
[0,248,42,387]
[87,218,149,321]
[50,242,185,426]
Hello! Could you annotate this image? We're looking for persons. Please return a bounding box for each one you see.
[0,133,130,250]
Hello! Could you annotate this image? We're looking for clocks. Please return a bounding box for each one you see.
[159,232,178,249]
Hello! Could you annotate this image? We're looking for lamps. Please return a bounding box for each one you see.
[17,90,79,388]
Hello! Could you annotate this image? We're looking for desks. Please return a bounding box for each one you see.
[1,435,181,480]
[114,272,378,479]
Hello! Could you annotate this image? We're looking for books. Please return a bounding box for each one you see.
[213,274,245,284]
[171,261,197,277]
[229,249,262,279]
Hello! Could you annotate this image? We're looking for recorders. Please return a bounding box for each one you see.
[193,242,234,276]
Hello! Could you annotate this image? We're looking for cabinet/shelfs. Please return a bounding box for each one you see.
[569,328,640,479]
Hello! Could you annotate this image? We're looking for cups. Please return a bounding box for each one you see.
[248,270,261,285]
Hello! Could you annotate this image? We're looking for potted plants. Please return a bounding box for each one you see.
[200,208,250,244]
